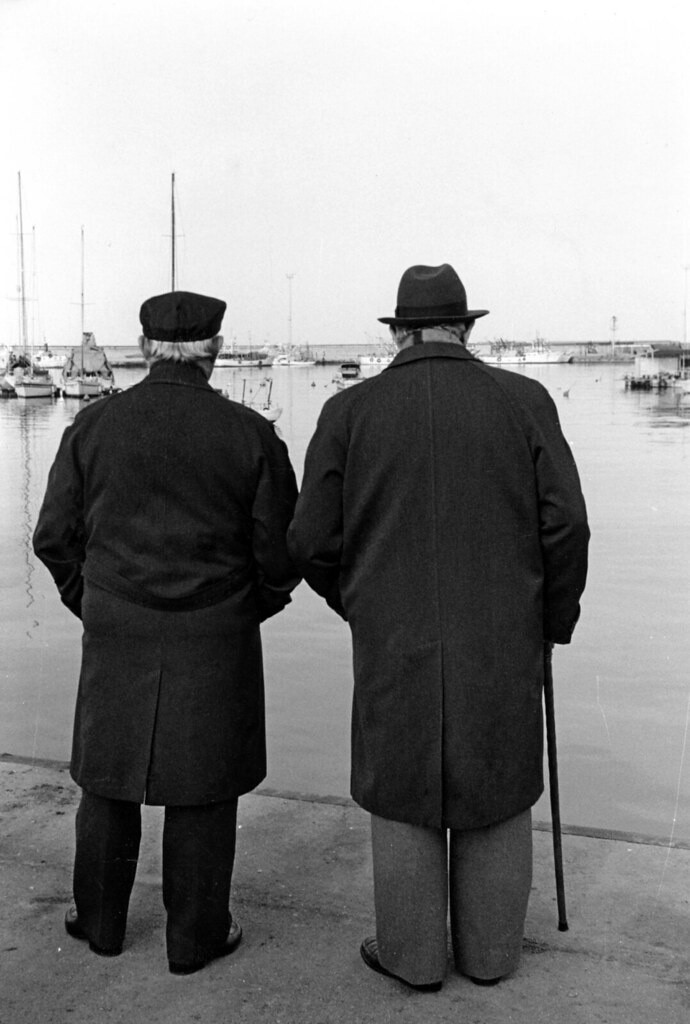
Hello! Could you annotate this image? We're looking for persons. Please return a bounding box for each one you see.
[33,292,300,975]
[285,262,592,990]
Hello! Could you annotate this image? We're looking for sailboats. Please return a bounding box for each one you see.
[111,173,277,367]
[4,170,67,398]
[60,225,104,399]
[273,279,316,365]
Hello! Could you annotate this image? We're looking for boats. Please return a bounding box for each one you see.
[479,338,573,364]
[357,349,481,365]
[335,363,367,390]
[571,315,635,365]
[214,377,283,423]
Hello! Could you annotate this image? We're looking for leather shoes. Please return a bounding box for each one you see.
[65,905,122,957]
[471,975,504,988]
[167,918,242,976]
[359,936,446,993]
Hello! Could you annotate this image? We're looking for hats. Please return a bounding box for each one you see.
[137,289,227,343]
[375,263,491,326]
